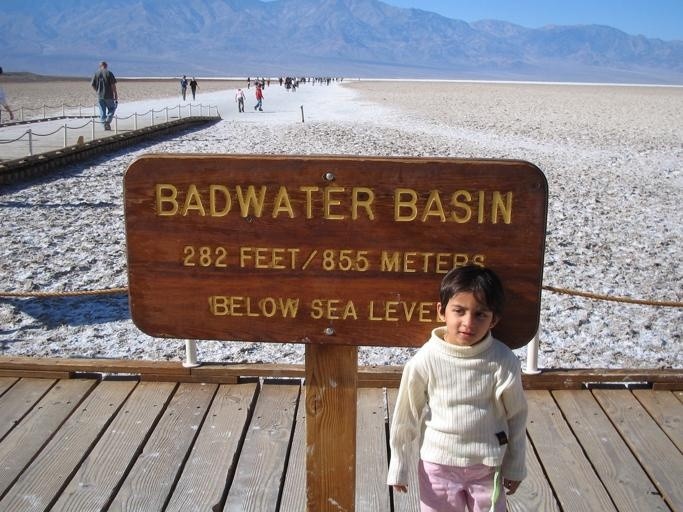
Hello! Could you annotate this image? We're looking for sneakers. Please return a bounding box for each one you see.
[104,121,111,130]
[259,107,263,111]
[255,106,257,110]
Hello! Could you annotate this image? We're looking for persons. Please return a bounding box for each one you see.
[246,74,344,91]
[386,262,529,512]
[187,75,199,101]
[252,84,263,113]
[234,88,245,113]
[88,61,118,130]
[0,67,13,123]
[177,74,190,101]
